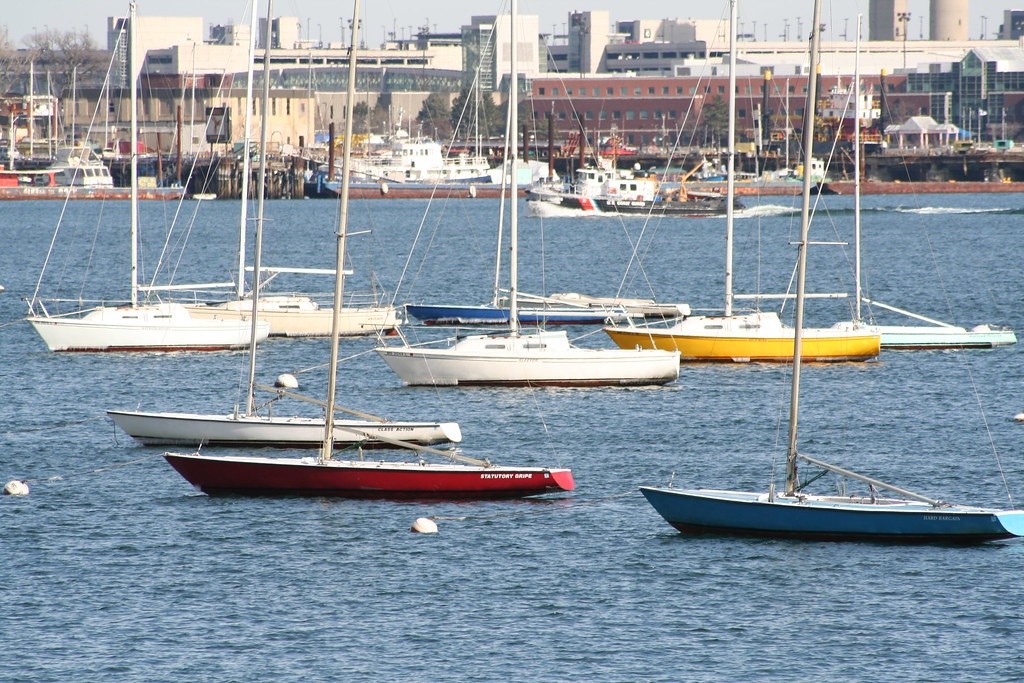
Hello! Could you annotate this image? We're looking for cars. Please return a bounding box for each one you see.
[592,144,637,155]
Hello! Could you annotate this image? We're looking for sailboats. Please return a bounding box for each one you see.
[161,1,576,499]
[105,0,463,449]
[25,1,1024,391]
[636,1,1024,544]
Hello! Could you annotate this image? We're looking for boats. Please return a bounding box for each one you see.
[523,122,745,218]
[0,144,188,200]
[322,131,540,199]
